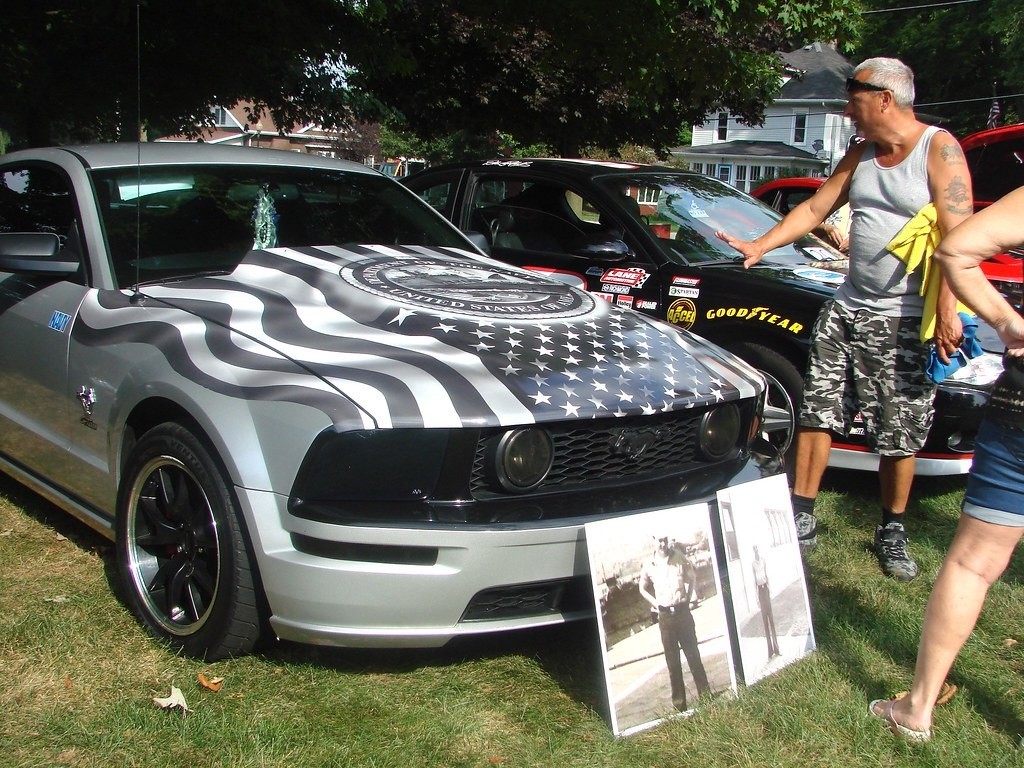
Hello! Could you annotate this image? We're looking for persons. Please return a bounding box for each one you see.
[638,531,713,711]
[751,543,782,662]
[868,184,1024,741]
[812,134,866,257]
[714,56,973,581]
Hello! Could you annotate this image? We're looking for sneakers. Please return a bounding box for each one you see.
[874,522,917,579]
[795,512,818,547]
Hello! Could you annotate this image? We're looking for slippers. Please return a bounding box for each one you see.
[868,693,930,745]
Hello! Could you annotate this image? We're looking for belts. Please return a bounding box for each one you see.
[659,605,682,613]
[758,584,767,588]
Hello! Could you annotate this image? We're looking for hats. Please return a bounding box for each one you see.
[651,529,667,540]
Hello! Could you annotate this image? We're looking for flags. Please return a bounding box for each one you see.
[987,90,999,130]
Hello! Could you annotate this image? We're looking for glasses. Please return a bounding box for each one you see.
[845,76,893,94]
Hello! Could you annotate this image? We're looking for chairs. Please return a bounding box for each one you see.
[488,210,525,248]
[270,198,319,237]
[599,196,641,227]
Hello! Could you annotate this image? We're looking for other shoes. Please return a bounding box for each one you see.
[774,651,782,656]
[768,652,774,660]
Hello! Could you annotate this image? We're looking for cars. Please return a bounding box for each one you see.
[393,161,1005,476]
[1,147,769,663]
[961,121,1024,284]
[675,177,829,251]
